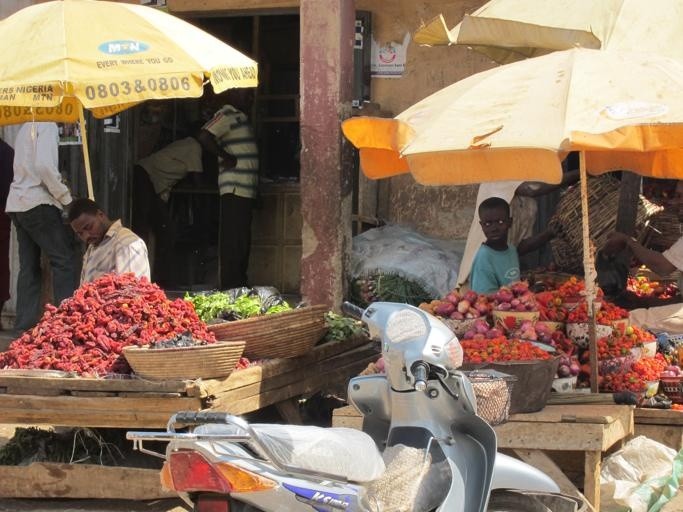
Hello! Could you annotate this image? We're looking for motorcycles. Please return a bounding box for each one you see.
[124,297,562,512]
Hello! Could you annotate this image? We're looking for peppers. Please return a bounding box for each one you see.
[0,272,249,376]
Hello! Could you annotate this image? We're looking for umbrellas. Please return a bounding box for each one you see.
[339,45,683,393]
[0,0,260,202]
[413,0,683,65]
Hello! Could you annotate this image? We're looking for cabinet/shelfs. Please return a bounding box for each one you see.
[164,17,299,291]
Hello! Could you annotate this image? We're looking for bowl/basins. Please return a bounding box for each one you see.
[438,311,683,414]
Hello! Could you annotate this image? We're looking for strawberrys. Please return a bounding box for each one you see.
[462,276,668,390]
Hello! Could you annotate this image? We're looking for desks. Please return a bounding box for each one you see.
[334,403,634,512]
[0,334,380,512]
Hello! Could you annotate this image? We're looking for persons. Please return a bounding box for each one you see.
[4,121,84,339]
[468,197,566,295]
[199,88,261,291]
[456,169,580,287]
[67,198,151,289]
[607,181,683,337]
[128,136,206,244]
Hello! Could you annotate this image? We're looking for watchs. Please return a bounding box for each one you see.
[628,237,637,250]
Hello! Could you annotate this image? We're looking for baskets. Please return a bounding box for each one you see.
[659,376,682,404]
[462,370,518,426]
[648,198,682,248]
[121,341,246,381]
[546,174,664,277]
[205,304,328,360]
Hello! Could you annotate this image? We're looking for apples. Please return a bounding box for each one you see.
[660,366,683,378]
[433,281,580,378]
